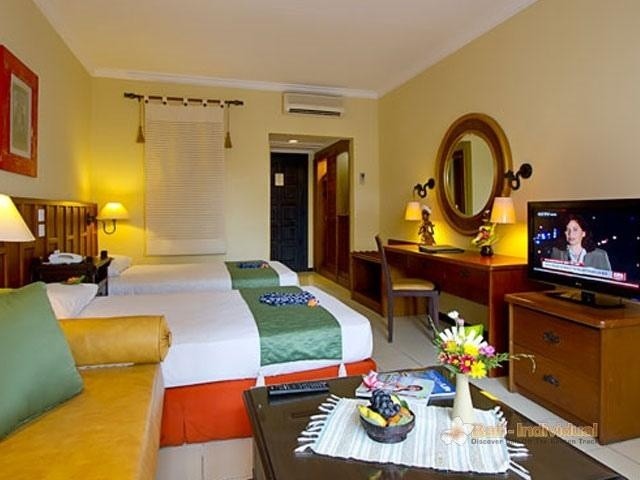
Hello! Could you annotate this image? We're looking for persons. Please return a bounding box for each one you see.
[418,207,436,245]
[379,381,423,392]
[552,215,613,269]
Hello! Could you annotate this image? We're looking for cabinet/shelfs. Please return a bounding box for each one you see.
[313,140,350,290]
[504,290,640,444]
[350,239,430,317]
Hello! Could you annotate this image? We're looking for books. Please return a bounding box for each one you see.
[418,244,465,254]
[377,368,457,399]
[353,371,435,408]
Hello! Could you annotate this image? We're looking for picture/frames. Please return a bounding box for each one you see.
[0,44,39,178]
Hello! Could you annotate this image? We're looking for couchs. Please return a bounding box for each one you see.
[0,314,172,480]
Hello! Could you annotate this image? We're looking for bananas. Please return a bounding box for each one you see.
[355,403,388,428]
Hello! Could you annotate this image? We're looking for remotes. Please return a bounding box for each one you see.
[268,380,329,396]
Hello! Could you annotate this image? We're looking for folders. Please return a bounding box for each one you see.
[419,245,465,253]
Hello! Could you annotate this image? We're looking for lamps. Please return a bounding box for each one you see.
[0,194,36,243]
[87,203,131,234]
[404,162,533,224]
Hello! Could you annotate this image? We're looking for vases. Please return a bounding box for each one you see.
[452,373,473,421]
[480,245,494,256]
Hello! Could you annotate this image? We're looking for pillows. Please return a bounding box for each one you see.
[0,280,99,441]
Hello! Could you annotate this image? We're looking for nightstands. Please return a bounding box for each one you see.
[32,252,113,296]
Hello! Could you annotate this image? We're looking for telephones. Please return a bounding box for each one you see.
[48,253,86,264]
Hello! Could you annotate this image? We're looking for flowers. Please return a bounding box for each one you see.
[471,210,501,247]
[432,311,536,378]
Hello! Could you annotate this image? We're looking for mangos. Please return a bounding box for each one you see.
[259,263,269,269]
[399,406,411,419]
[388,414,402,425]
[307,299,317,307]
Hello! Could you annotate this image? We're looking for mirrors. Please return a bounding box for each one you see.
[434,112,514,236]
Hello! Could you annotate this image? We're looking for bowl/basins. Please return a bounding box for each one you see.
[358,407,417,445]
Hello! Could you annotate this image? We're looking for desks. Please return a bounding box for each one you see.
[383,244,535,378]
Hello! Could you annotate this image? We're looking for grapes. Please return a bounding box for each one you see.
[235,259,267,269]
[257,290,317,306]
[369,385,401,419]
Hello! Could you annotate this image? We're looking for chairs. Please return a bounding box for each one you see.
[374,236,441,343]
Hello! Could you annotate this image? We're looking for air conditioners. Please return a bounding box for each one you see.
[282,93,344,119]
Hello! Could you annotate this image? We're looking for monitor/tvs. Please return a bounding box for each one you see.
[527,199,640,311]
